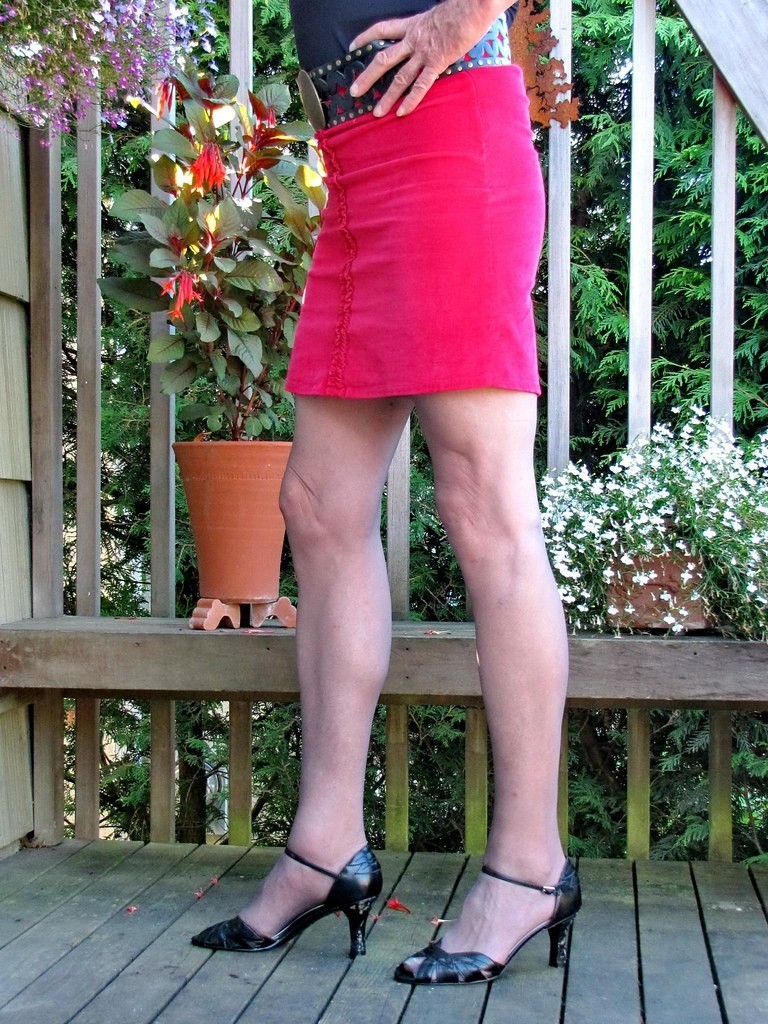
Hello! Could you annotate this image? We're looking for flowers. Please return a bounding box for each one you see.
[0,0,220,149]
[535,397,768,643]
[97,64,327,439]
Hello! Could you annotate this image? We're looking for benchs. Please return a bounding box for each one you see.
[0,616,768,702]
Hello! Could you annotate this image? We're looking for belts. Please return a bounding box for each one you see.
[298,12,514,133]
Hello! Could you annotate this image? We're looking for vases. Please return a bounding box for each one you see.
[604,522,704,632]
[174,440,294,632]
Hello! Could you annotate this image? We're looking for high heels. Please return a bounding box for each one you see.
[190,843,384,957]
[393,858,582,985]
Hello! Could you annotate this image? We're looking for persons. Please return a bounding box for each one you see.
[190,0,584,987]
